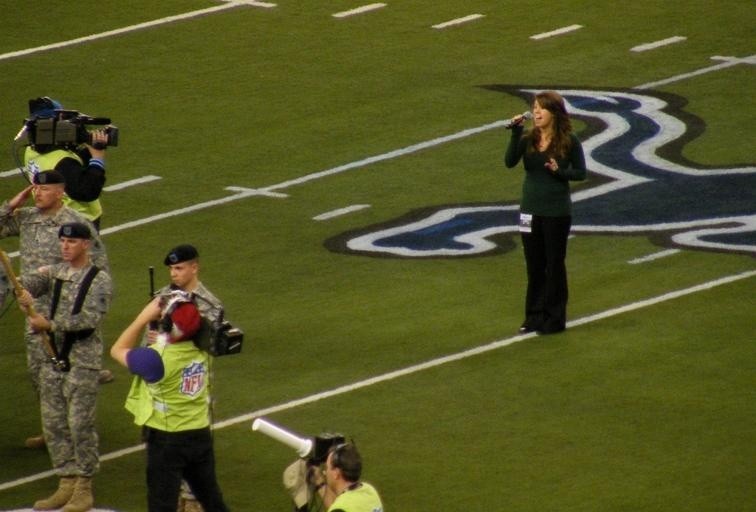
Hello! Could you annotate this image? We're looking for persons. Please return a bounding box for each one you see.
[290,441,383,512]
[22,97,115,383]
[0,169,112,448]
[108,290,228,511]
[147,242,227,512]
[501,90,588,339]
[5,225,116,511]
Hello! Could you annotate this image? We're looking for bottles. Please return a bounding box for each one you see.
[28,96,62,120]
[163,244,199,265]
[165,303,202,344]
[59,222,91,239]
[33,169,65,184]
[283,458,314,509]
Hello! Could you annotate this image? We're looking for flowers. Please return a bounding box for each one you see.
[505,111,535,128]
[88,117,111,125]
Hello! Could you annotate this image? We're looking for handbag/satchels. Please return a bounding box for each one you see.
[313,480,327,491]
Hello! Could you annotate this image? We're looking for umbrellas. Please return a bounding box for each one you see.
[519,322,531,333]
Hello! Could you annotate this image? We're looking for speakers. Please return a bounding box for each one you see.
[33,478,76,509]
[60,476,94,512]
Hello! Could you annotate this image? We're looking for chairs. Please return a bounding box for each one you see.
[98,370,114,384]
[25,433,47,449]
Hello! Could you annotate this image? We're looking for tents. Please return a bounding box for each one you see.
[161,298,194,334]
